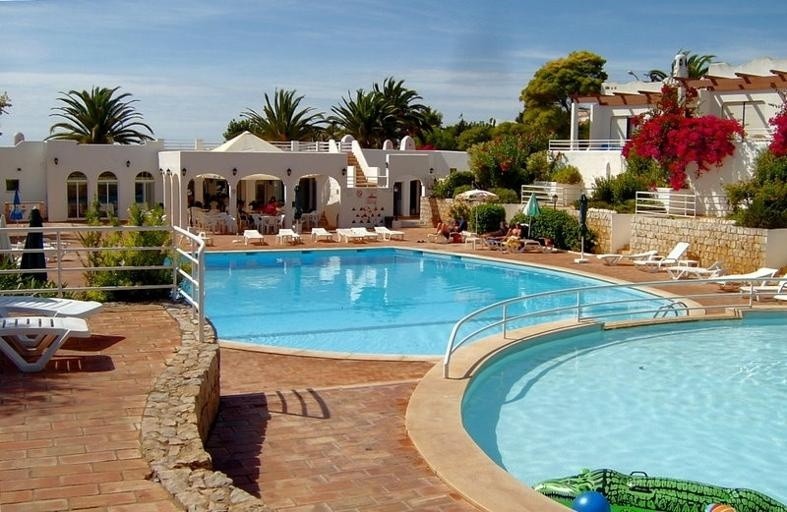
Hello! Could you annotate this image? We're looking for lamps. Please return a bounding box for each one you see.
[159,167,187,176]
[233,168,237,176]
[342,168,346,176]
[429,168,434,174]
[287,168,291,176]
[126,160,131,168]
[54,158,58,165]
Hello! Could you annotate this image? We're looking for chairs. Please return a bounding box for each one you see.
[274,226,302,246]
[0,315,90,373]
[310,226,334,243]
[0,296,103,347]
[336,225,406,243]
[425,222,555,257]
[11,207,71,266]
[594,240,785,302]
[240,228,266,247]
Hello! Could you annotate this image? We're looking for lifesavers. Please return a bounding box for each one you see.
[533,468,787,511]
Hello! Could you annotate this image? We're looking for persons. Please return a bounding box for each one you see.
[434,220,460,239]
[473,219,506,239]
[189,193,304,233]
[486,221,522,242]
[455,214,467,234]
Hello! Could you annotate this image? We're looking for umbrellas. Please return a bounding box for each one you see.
[455,188,499,237]
[19,205,48,285]
[521,190,542,238]
[577,192,589,258]
[9,191,23,225]
[0,215,15,265]
